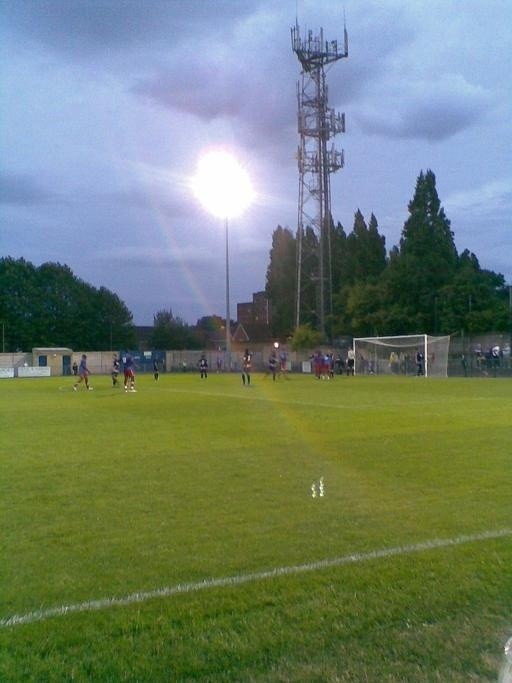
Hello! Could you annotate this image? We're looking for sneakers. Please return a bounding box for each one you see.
[72,386,77,391]
[87,387,93,390]
[123,386,136,393]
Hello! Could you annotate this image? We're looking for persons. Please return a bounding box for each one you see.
[153,359,159,380]
[72,354,93,391]
[309,345,377,380]
[112,348,137,392]
[390,351,436,376]
[73,362,78,375]
[242,349,252,384]
[264,346,287,380]
[197,355,208,379]
[473,343,511,368]
